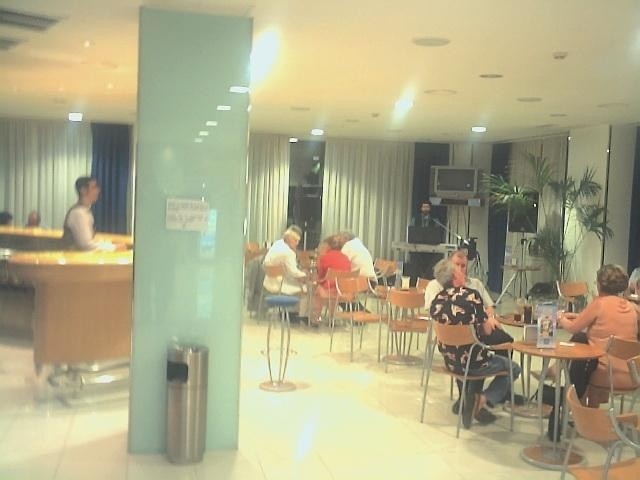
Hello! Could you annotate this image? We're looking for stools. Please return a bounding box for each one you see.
[259,295,299,392]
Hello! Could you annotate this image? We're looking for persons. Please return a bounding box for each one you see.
[408,201,442,228]
[623,268,640,305]
[337,232,378,324]
[430,261,522,424]
[0,211,13,228]
[424,250,530,414]
[27,211,41,230]
[64,176,130,252]
[261,230,312,325]
[309,237,352,328]
[530,263,640,443]
[288,225,303,237]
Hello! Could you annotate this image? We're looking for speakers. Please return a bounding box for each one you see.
[508,191,539,233]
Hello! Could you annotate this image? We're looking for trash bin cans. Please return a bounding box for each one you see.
[164,345,209,464]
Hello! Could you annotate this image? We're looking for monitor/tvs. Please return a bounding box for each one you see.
[434,165,478,200]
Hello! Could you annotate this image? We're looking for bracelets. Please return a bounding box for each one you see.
[556,313,564,323]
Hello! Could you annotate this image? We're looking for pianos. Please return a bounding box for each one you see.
[393,243,456,253]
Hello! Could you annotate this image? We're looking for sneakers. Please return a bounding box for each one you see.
[452,397,461,415]
[474,407,497,424]
[507,392,529,407]
[460,391,482,429]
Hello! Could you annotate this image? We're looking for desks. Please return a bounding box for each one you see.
[503,265,542,301]
[496,313,563,418]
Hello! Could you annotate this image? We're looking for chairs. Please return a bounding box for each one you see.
[308,267,360,326]
[560,384,640,479]
[581,334,640,414]
[617,354,640,460]
[420,318,515,438]
[556,280,587,313]
[256,263,284,325]
[408,277,431,350]
[330,276,388,361]
[245,242,266,319]
[385,287,425,373]
[374,258,397,285]
[512,341,606,470]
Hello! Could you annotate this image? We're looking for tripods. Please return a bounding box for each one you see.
[494,238,528,304]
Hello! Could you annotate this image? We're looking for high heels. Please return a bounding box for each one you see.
[529,368,557,387]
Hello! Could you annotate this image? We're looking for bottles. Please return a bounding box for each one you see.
[523,294,535,326]
